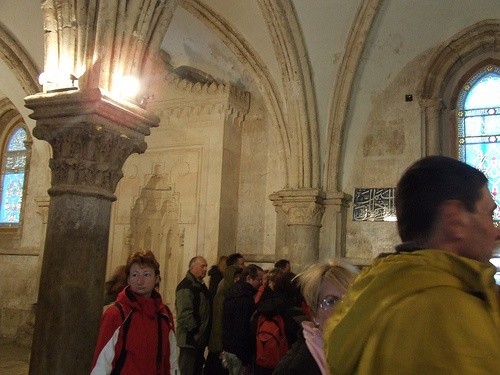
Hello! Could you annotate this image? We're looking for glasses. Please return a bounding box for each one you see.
[318,295,341,311]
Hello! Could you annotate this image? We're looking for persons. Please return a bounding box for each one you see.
[100,264,129,320]
[272,260,364,375]
[324,156,500,375]
[175,254,316,375]
[86,248,182,375]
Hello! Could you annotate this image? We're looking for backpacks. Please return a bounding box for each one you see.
[255,303,287,369]
